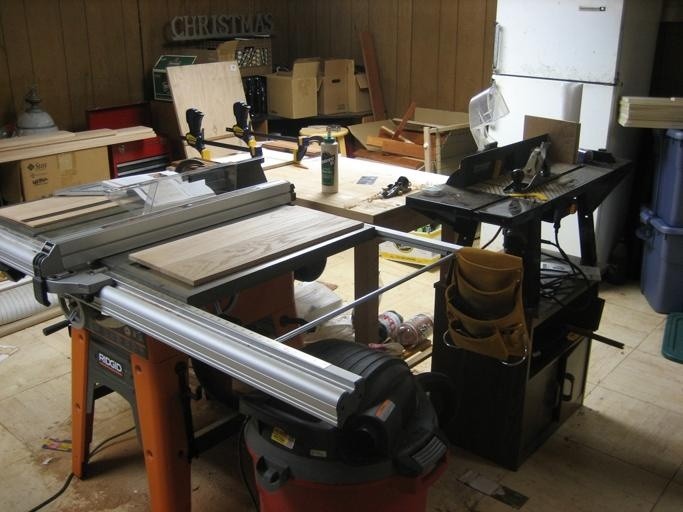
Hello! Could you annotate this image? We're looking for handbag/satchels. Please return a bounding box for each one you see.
[442,247,533,366]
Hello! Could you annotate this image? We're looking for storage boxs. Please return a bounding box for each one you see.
[149,37,372,160]
[635,127,683,314]
[0,146,111,203]
[347,107,478,176]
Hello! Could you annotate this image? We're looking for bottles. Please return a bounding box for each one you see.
[321,127,339,194]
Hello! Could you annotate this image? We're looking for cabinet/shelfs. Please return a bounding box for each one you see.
[429,247,611,472]
